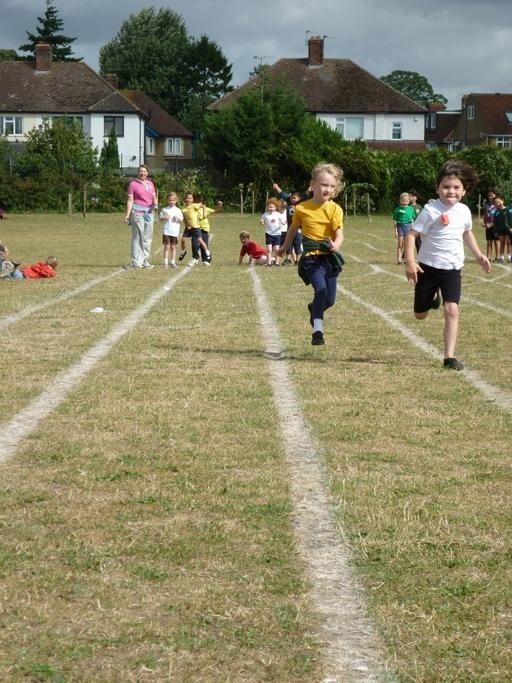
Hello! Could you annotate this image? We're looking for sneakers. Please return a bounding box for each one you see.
[444,358,462,370]
[312,331,323,345]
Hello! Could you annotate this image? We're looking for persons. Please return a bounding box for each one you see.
[273,162,346,347]
[2,254,59,278]
[481,191,512,263]
[234,182,313,265]
[404,161,493,371]
[393,188,424,263]
[125,161,223,269]
